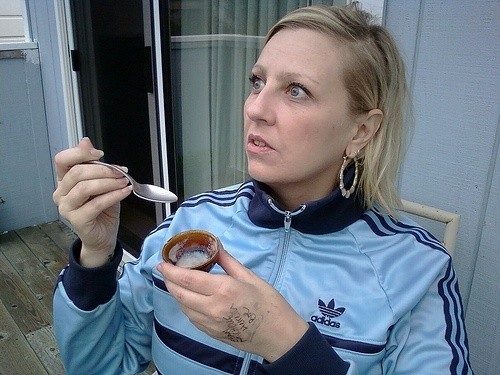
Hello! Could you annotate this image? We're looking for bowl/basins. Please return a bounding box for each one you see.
[162,229,219,273]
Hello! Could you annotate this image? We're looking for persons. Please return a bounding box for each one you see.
[52,0,475,375]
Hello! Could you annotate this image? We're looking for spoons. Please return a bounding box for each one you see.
[85,160,179,203]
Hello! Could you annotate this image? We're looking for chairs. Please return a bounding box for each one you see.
[152,197,461,375]
